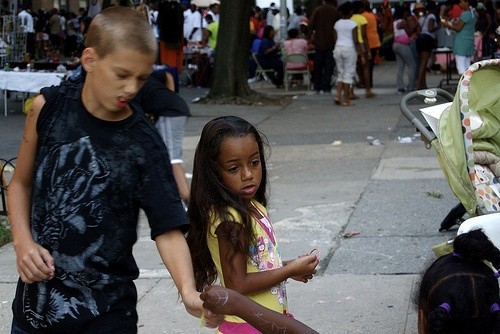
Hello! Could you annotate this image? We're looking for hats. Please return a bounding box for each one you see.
[412,3,426,14]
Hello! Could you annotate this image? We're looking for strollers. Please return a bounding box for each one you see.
[400,59,500,252]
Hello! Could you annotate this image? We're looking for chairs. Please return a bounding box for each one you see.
[251,51,311,91]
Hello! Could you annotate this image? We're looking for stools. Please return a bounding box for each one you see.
[435,47,453,85]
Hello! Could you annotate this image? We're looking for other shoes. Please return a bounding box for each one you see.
[350,94,358,99]
[398,88,407,95]
[365,93,375,98]
[334,95,340,105]
[343,101,350,106]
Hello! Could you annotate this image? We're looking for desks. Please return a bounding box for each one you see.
[0,70,68,117]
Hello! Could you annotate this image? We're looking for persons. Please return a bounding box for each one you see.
[133,66,191,210]
[178,115,321,334]
[6,4,227,334]
[0,0,500,106]
[200,228,500,334]
